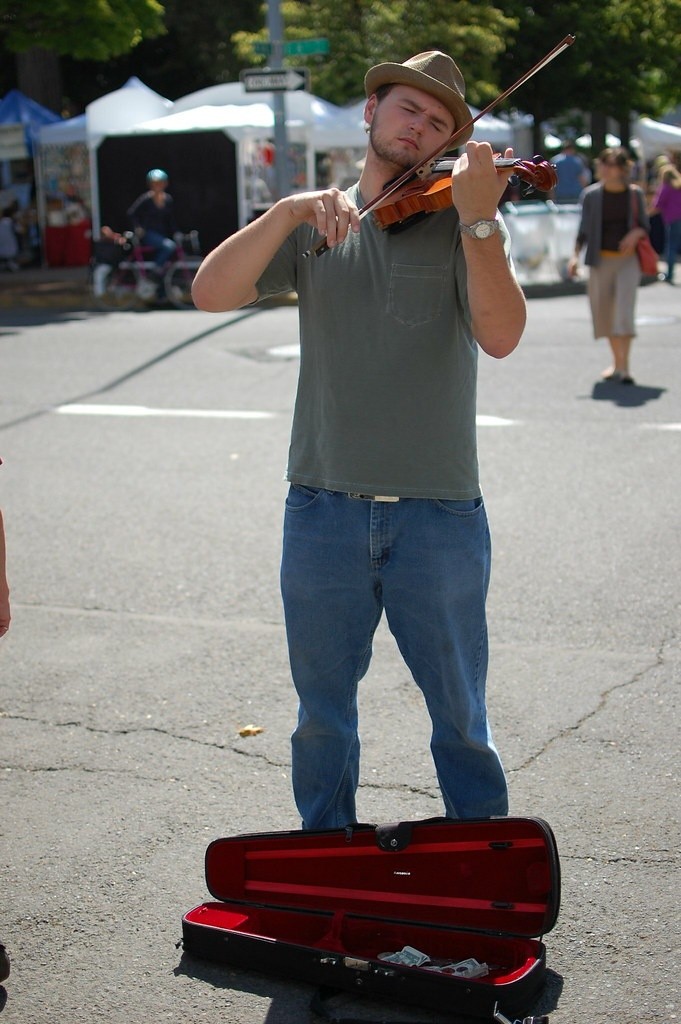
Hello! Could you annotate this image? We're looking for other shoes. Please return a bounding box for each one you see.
[613,369,633,384]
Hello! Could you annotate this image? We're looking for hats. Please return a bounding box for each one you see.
[365,51,474,151]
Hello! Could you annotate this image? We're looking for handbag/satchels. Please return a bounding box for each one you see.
[630,183,657,278]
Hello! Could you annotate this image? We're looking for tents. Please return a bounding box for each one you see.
[512,109,681,160]
[0,76,511,269]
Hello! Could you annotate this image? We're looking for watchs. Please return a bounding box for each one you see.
[459,219,500,241]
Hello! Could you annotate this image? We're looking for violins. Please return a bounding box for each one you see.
[369,151,559,232]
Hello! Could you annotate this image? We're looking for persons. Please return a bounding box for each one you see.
[92,169,183,300]
[0,457,13,983]
[567,148,651,385]
[548,140,681,282]
[0,195,91,277]
[191,51,526,829]
